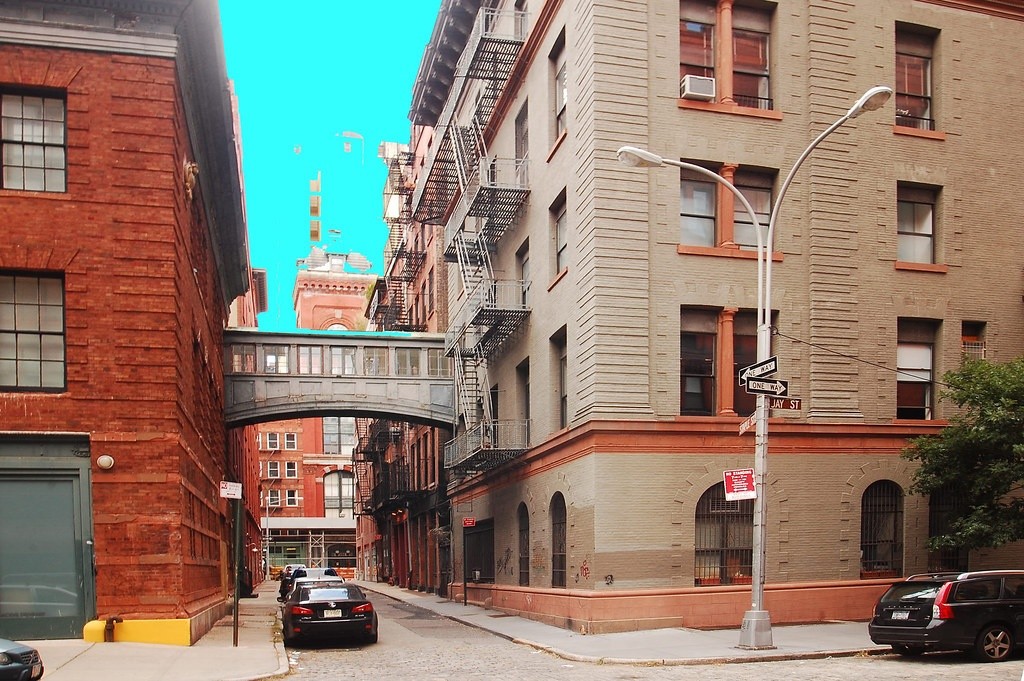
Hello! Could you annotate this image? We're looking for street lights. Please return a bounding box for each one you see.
[618,84,896,651]
[265,496,271,581]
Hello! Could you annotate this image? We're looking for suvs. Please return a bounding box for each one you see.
[870,569,1024,662]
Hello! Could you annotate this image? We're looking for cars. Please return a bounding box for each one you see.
[281,564,345,602]
[0,638,45,681]
[277,583,379,644]
